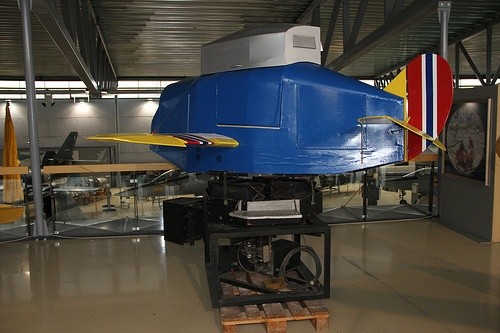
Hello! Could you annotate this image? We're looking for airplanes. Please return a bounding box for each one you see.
[0,131,100,183]
[85,23,454,175]
